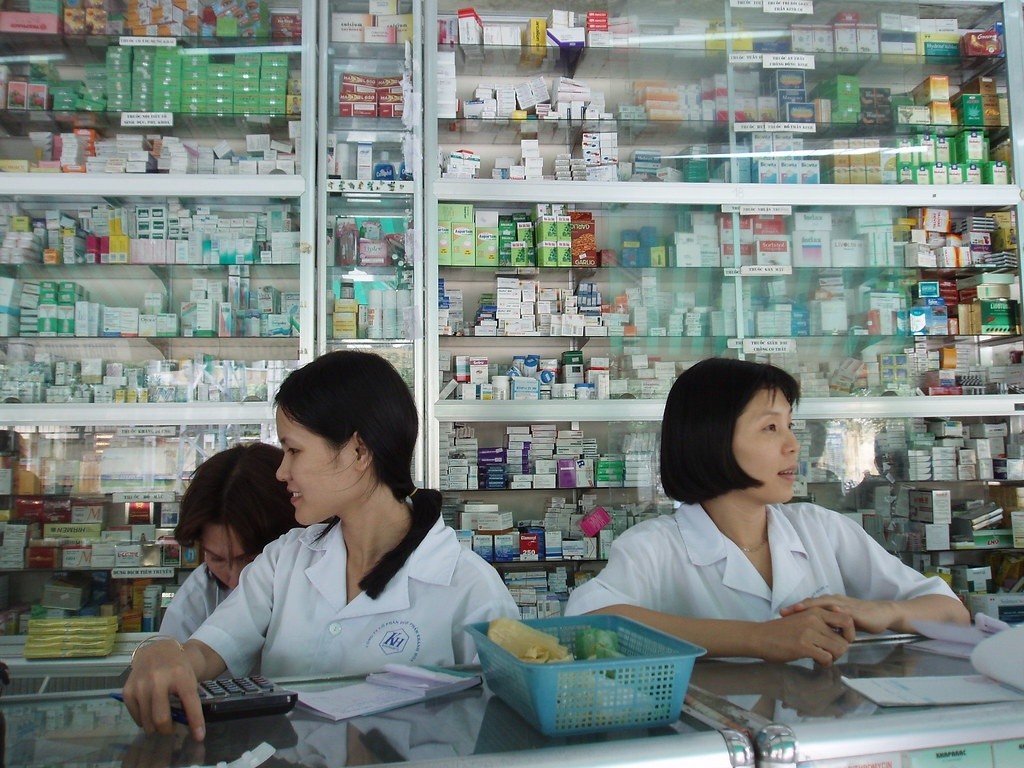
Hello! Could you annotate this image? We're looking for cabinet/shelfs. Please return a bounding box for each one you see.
[0,632,1024,766]
[315,0,425,493]
[0,1,318,573]
[424,1,1022,569]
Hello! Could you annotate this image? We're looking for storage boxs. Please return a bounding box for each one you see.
[0,0,1024,632]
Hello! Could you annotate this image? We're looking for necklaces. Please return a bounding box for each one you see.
[740,541,767,552]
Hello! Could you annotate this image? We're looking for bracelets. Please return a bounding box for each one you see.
[130,635,183,661]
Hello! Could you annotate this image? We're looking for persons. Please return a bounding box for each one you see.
[158,443,333,680]
[563,357,971,667]
[124,352,520,742]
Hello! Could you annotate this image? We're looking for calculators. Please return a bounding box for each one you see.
[109,671,301,727]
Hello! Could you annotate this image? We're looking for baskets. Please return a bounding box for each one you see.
[465,613,708,737]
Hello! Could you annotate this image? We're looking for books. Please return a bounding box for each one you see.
[841,613,1024,706]
[280,660,484,722]
